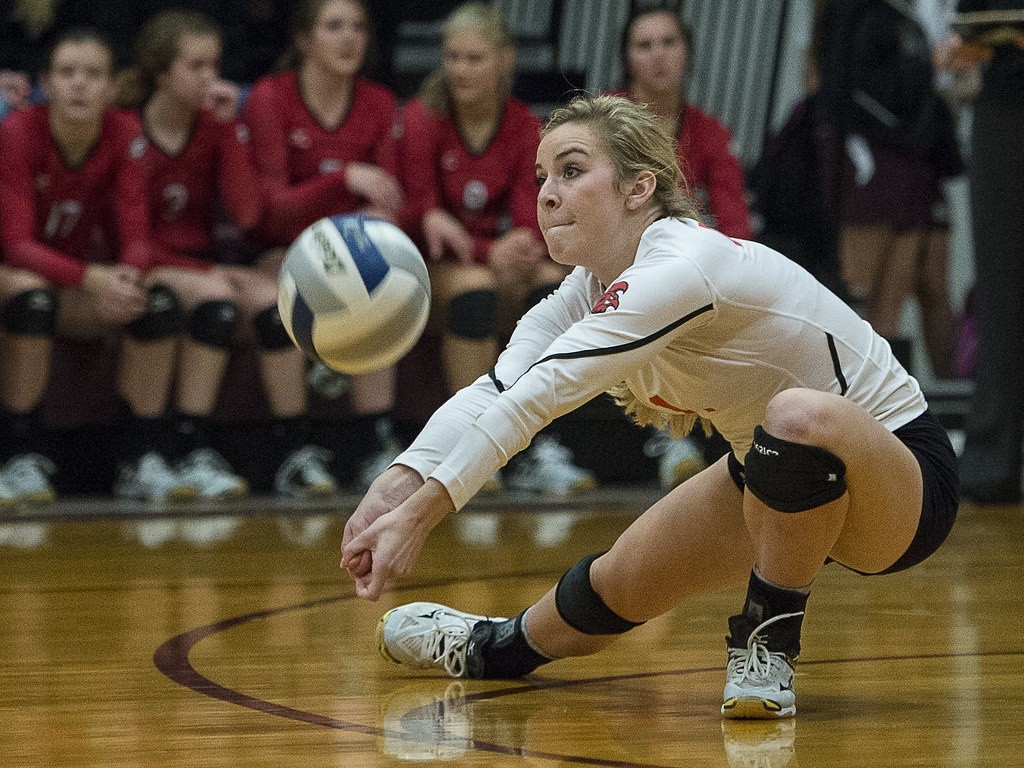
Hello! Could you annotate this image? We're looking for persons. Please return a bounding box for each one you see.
[0,0,1024,512]
[341,95,961,720]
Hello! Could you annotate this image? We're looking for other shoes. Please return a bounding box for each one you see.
[645,433,706,489]
[359,418,400,489]
[483,471,503,491]
[0,472,22,505]
[176,447,249,499]
[112,449,198,502]
[507,434,596,493]
[274,445,337,498]
[7,450,59,502]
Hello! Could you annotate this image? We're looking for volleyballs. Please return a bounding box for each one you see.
[276,213,432,376]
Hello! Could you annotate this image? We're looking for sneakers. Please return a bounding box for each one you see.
[720,610,804,717]
[720,721,796,768]
[375,602,535,679]
[379,681,558,760]
